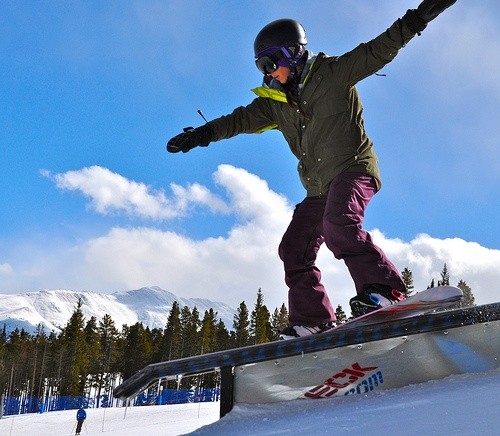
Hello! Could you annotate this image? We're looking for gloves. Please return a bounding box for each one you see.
[418,0,457,23]
[166,126,208,153]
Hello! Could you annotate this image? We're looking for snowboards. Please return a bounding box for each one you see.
[321,286,464,334]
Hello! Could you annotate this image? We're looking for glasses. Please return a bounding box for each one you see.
[255,53,279,74]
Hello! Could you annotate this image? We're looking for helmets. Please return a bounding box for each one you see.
[80,405,84,409]
[253,18,308,55]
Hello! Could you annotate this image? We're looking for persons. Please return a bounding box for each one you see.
[167,0,458,340]
[73,407,87,434]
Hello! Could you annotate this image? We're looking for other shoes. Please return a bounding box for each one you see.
[78,433,80,435]
[75,433,77,435]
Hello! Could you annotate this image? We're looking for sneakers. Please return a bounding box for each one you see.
[348,287,407,318]
[279,321,337,341]
